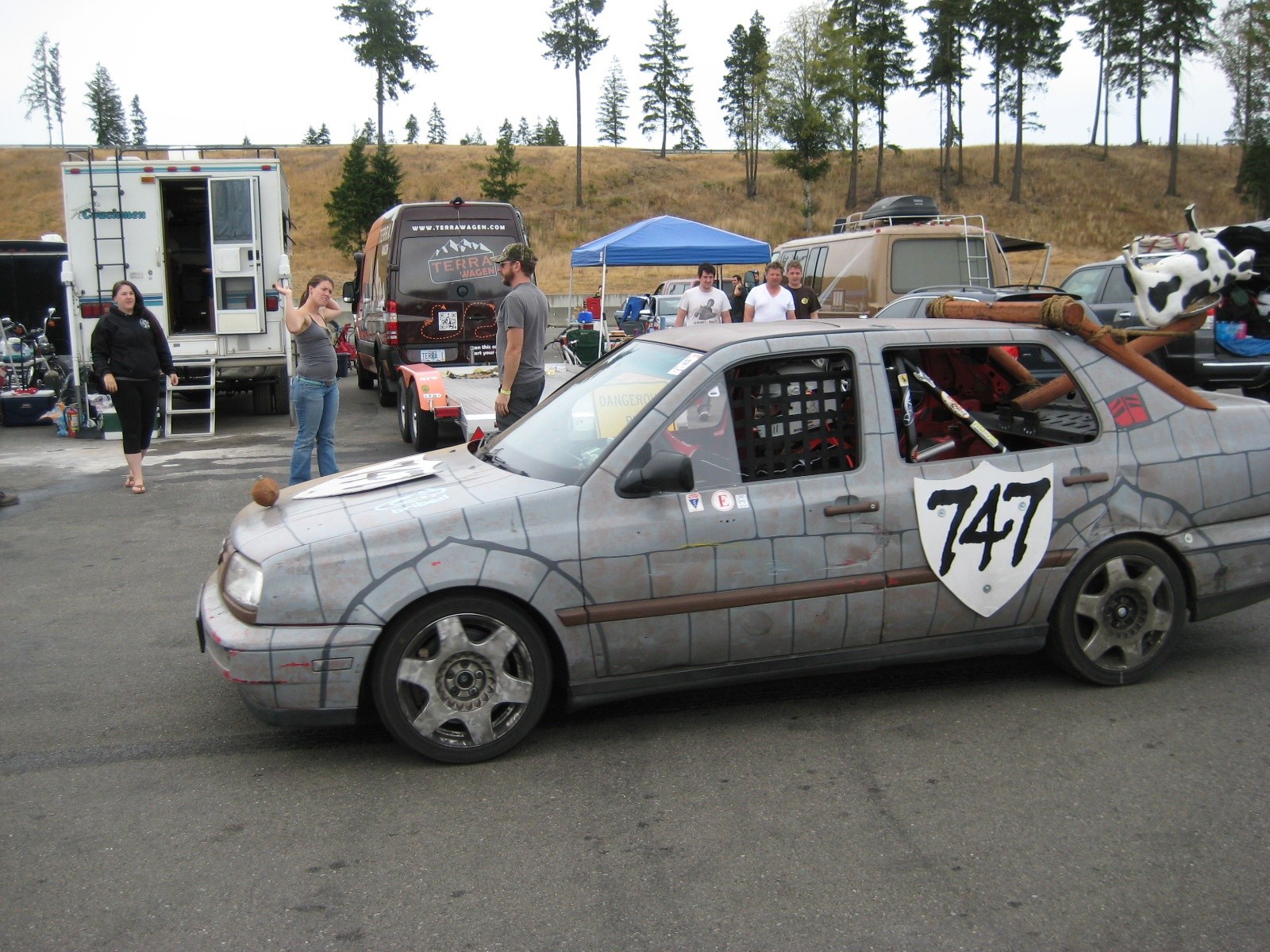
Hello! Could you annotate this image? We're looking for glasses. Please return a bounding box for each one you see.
[499,261,515,265]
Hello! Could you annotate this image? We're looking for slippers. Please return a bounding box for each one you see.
[125,476,135,486]
[131,485,146,493]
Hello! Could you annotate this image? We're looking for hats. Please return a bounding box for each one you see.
[488,242,538,265]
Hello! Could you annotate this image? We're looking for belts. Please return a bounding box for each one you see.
[296,374,337,386]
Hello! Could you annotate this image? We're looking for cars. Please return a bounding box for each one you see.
[613,294,687,335]
[194,322,1270,762]
[326,321,359,376]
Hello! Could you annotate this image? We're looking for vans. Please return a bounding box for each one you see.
[342,198,534,407]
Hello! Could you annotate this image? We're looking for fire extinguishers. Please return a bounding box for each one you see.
[64,402,79,437]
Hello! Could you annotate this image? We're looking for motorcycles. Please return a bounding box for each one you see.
[0,307,71,399]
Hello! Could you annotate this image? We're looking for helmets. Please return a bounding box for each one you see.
[752,362,837,438]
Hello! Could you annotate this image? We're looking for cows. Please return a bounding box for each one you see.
[1124,203,1261,329]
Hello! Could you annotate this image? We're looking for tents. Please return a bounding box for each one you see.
[568,215,772,359]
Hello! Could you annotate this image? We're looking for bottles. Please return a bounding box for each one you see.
[1215,320,1236,342]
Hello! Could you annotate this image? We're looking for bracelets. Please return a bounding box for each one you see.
[500,388,511,395]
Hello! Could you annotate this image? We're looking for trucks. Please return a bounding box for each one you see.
[0,234,69,422]
[61,149,299,416]
[743,194,1052,365]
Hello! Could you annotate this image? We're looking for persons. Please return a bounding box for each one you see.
[91,281,178,494]
[488,243,550,432]
[675,263,733,327]
[274,274,344,486]
[732,260,822,323]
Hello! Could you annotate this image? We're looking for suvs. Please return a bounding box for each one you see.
[1053,256,1269,402]
[860,284,1106,360]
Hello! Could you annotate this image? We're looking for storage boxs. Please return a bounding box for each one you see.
[0,389,56,426]
[102,407,162,440]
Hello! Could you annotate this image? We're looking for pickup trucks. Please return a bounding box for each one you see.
[643,278,735,296]
